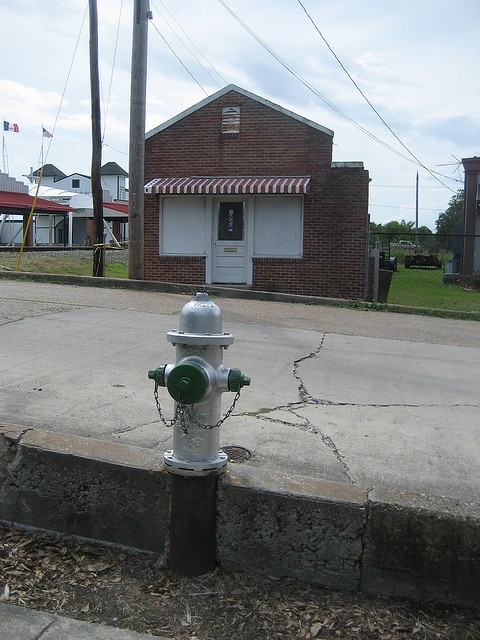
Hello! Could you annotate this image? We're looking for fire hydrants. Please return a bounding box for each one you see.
[148,292,251,478]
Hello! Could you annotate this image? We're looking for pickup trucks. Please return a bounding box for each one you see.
[390,240,416,249]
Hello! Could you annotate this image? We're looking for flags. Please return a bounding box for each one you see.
[4,121,19,132]
[43,128,53,137]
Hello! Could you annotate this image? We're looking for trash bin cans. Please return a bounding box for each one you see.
[367,269,394,303]
